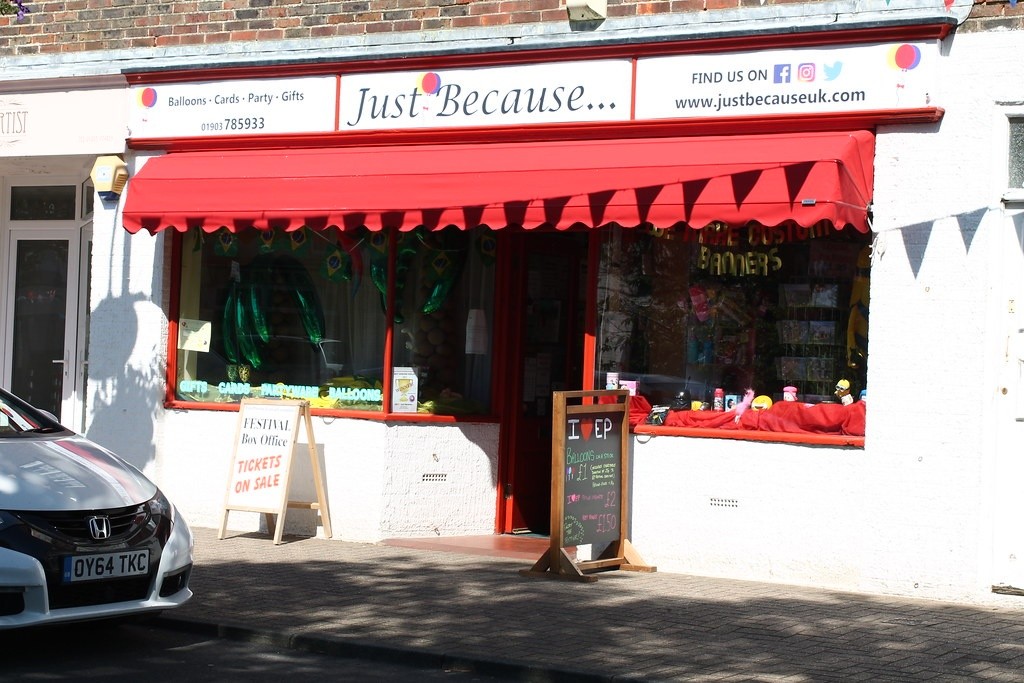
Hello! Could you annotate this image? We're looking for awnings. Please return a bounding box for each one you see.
[120,124,884,237]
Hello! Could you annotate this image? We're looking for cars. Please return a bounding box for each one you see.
[0,388,193,628]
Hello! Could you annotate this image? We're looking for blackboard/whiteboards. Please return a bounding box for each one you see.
[553,390,632,549]
[225,400,305,515]
